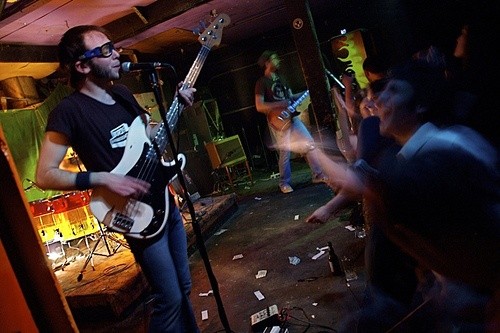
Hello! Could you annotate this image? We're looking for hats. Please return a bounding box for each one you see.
[257,49,280,69]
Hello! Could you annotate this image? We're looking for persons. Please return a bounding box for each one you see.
[254,50,329,194]
[34,25,200,333]
[272,21,500,333]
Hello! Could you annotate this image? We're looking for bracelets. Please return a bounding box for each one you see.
[302,144,318,157]
[76,172,94,191]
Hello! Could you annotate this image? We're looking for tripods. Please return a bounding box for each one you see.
[77,220,131,282]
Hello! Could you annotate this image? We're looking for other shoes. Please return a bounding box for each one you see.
[279,182,293,193]
[312,178,328,183]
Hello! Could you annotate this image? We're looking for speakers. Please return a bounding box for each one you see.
[204,134,247,171]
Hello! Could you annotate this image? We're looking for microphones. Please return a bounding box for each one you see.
[121,62,170,71]
[27,179,45,192]
[227,151,234,154]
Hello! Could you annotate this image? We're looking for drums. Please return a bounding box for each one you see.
[27,198,60,245]
[48,187,106,242]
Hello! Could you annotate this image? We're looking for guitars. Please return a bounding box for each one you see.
[89,9,232,239]
[267,90,309,130]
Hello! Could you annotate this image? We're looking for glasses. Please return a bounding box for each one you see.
[78,41,115,61]
